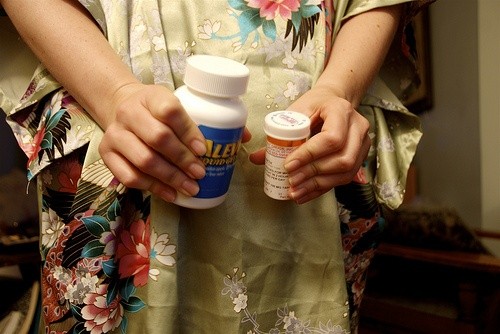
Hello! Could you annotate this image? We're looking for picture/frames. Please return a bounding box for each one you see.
[387,4,434,118]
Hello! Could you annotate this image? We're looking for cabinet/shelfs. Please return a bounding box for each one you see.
[358,240,500,334]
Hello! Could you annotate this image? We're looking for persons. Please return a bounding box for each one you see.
[0,0,440,334]
